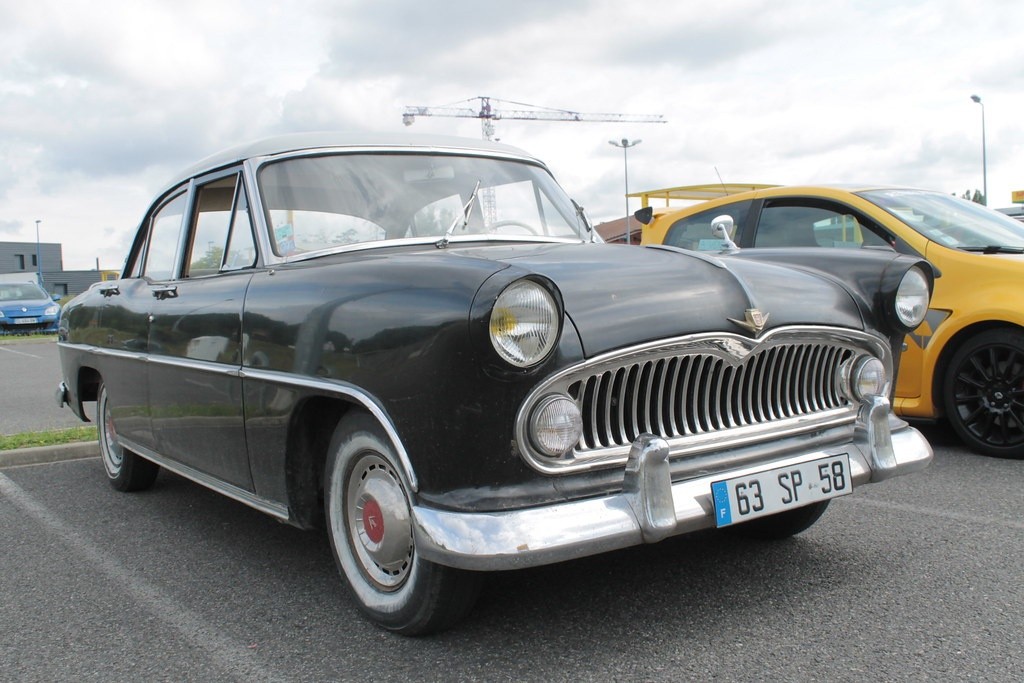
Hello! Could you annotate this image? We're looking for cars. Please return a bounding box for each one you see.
[626,183,1023,458]
[57,132,897,634]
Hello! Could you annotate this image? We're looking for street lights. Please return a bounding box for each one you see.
[36,219,43,286]
[971,95,988,208]
[607,138,643,244]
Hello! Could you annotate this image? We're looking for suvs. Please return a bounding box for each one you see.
[0,280,62,334]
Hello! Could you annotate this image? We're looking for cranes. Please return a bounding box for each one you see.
[400,96,668,235]
